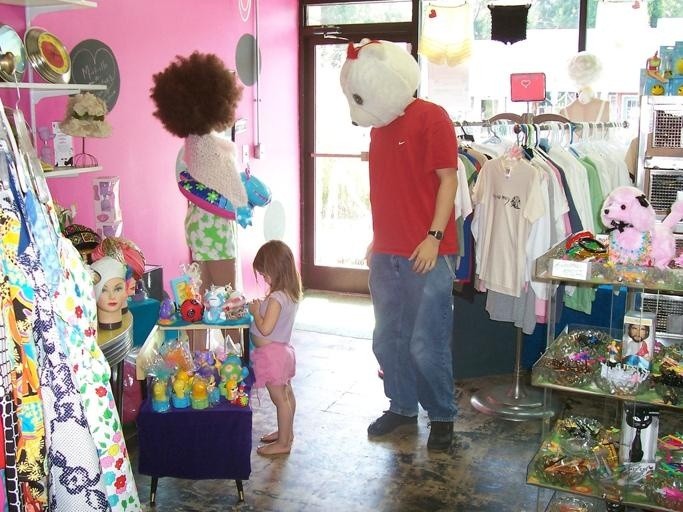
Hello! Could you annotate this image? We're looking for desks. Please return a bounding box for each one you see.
[159,311,254,366]
[138,398,252,508]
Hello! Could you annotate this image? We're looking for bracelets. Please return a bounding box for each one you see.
[579,237,607,253]
[565,232,595,251]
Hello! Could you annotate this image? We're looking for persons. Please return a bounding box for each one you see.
[152,49,249,353]
[341,36,465,451]
[645,49,670,84]
[88,255,128,330]
[245,238,304,458]
[557,50,620,140]
[624,323,652,362]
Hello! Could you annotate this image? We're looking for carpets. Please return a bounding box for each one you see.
[293,289,373,341]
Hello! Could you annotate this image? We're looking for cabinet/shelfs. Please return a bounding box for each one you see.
[525,232,682,512]
[0,0,109,180]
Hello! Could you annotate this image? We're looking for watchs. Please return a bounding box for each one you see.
[426,228,444,242]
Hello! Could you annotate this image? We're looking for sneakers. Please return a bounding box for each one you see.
[427,421,453,449]
[368,411,418,435]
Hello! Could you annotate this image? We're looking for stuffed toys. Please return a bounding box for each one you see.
[601,184,683,270]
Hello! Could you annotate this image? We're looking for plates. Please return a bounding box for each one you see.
[0,24,27,83]
[23,25,72,86]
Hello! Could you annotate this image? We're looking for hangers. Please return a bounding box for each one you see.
[456,119,624,160]
